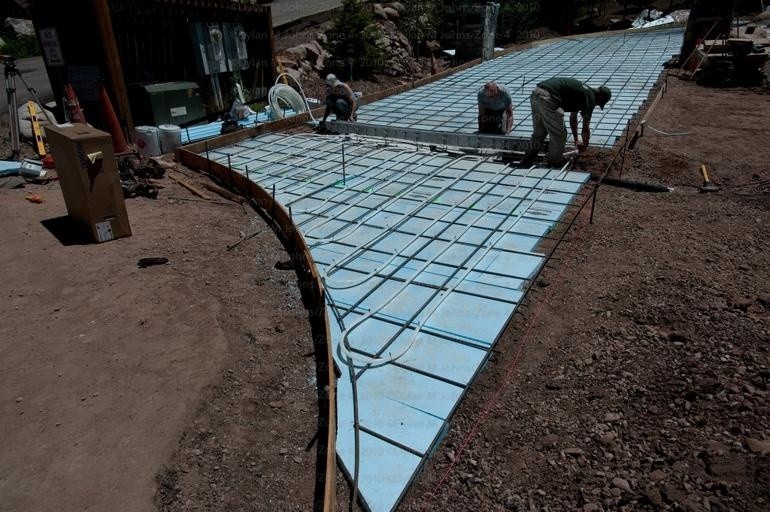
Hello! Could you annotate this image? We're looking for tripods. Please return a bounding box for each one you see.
[0,64,55,162]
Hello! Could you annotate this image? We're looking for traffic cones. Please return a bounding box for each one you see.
[101,84,131,156]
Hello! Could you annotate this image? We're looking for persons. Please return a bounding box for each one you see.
[477,82,513,136]
[518,77,611,168]
[319,74,359,126]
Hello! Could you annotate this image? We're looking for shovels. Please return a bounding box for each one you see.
[700,165,719,193]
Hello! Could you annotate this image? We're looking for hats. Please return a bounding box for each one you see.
[326,73,341,88]
[482,82,498,97]
[598,86,612,111]
